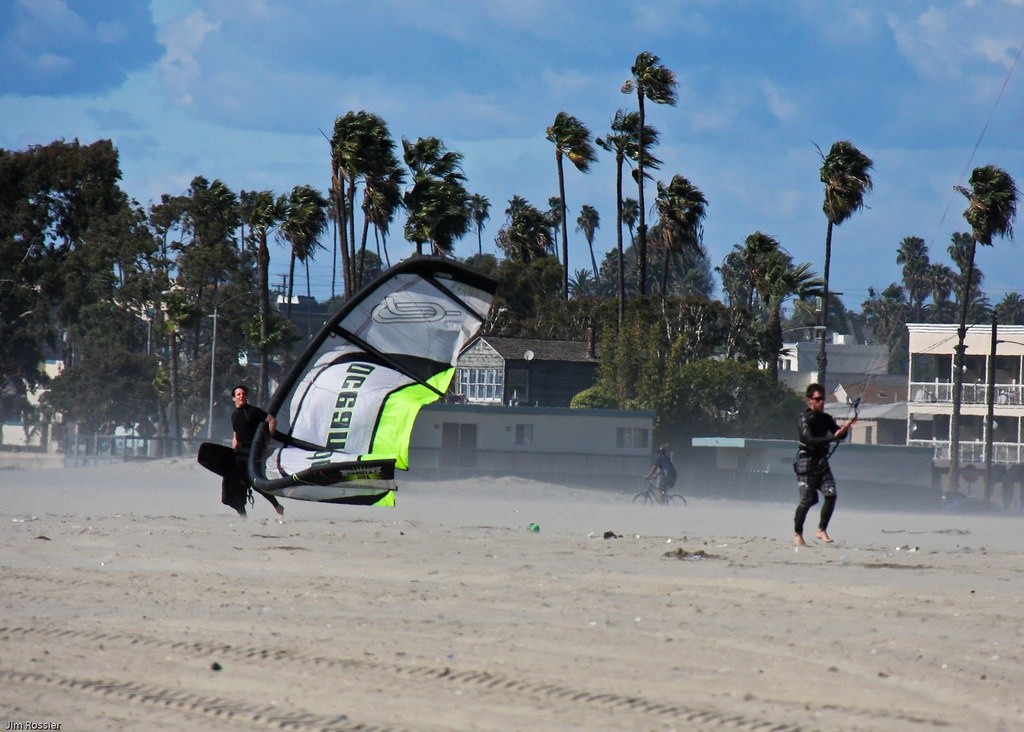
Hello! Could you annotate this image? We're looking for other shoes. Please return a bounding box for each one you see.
[817,530,833,543]
[793,534,808,548]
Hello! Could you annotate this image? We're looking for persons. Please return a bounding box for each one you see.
[793,383,853,548]
[645,444,677,504]
[231,386,285,520]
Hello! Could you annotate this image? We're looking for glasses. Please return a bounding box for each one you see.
[811,396,824,402]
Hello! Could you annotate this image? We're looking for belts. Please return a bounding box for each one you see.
[799,445,811,451]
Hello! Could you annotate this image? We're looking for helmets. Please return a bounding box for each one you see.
[656,447,665,453]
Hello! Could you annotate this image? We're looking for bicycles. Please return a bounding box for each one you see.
[633,476,687,508]
